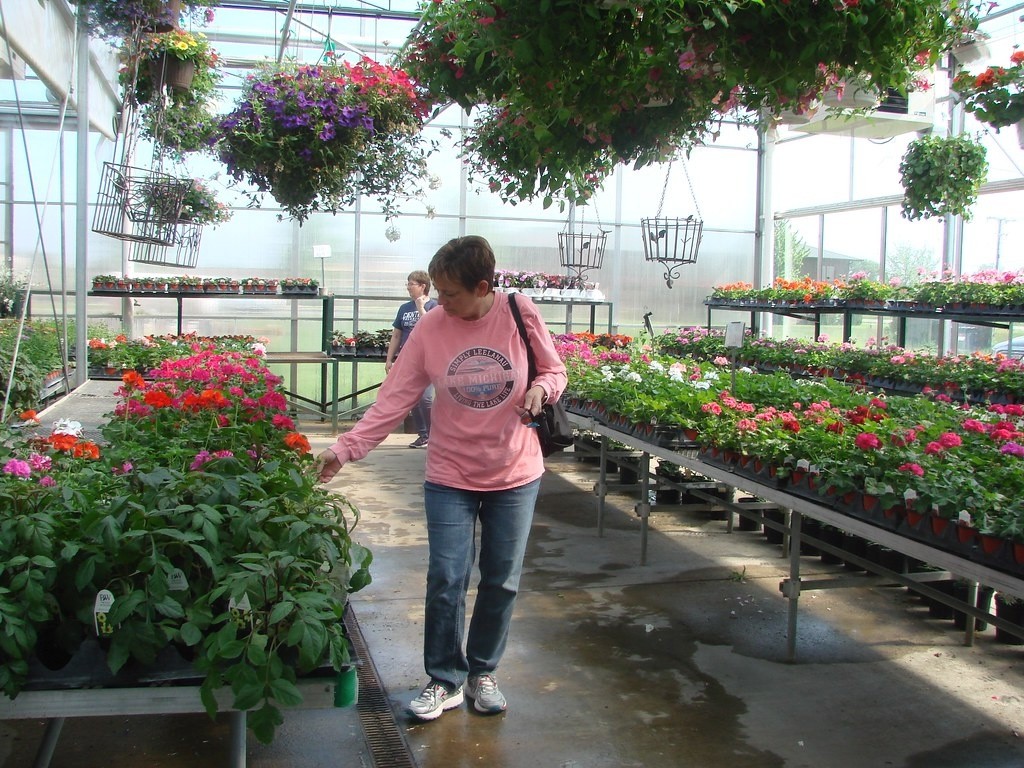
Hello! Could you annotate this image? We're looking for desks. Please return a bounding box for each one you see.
[0,378,359,768]
[564,410,1024,660]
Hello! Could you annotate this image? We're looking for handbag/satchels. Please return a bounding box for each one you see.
[508,293,574,458]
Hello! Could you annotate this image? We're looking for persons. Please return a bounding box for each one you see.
[309,235,569,721]
[386,271,438,448]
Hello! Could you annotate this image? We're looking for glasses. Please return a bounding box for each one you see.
[405,283,421,287]
[525,409,540,428]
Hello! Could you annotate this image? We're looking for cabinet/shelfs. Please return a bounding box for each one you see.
[88,292,613,437]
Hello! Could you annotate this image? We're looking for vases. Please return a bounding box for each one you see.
[777,105,812,125]
[571,300,1024,566]
[822,74,878,108]
[94,283,317,291]
[950,41,992,66]
[147,56,194,94]
[493,286,605,301]
[1016,117,1024,149]
[139,0,181,34]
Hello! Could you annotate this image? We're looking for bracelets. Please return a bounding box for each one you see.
[541,392,547,404]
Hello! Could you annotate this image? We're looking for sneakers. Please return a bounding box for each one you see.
[466,673,507,713]
[409,436,429,448]
[406,681,464,720]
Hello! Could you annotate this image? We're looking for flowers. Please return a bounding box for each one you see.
[498,325,1024,548]
[92,275,319,286]
[493,271,600,290]
[68,0,1024,222]
[0,332,379,768]
[711,263,1024,306]
